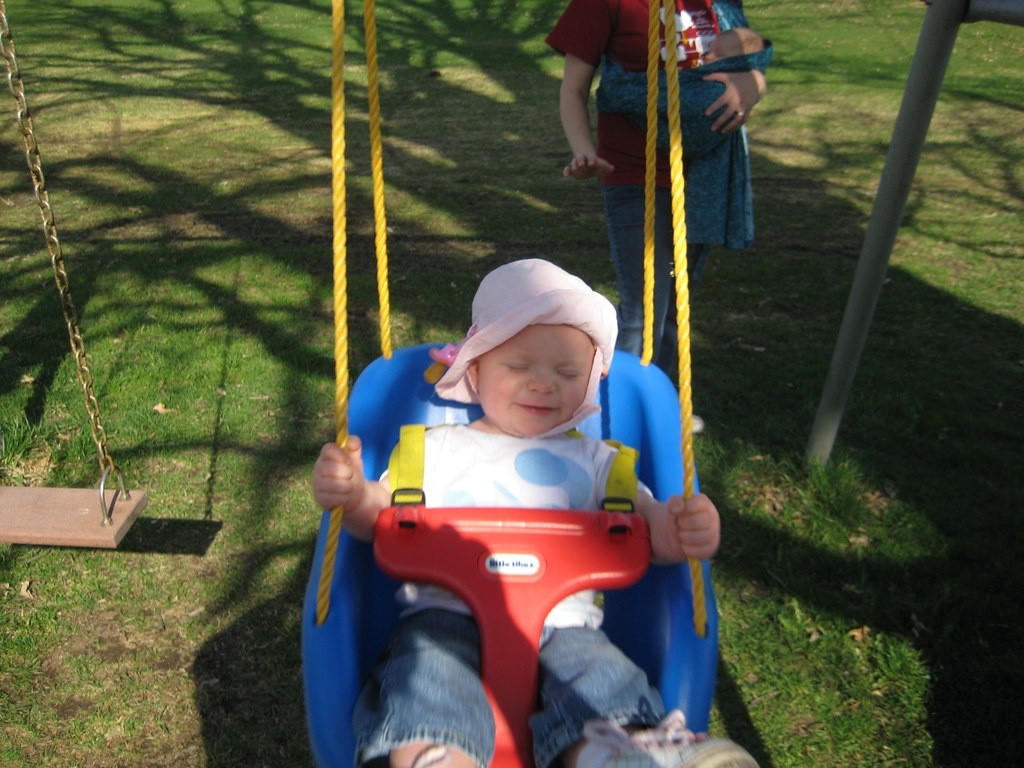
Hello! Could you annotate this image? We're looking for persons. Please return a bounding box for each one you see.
[312,258,757,768]
[544,0,772,433]
[596,26,772,161]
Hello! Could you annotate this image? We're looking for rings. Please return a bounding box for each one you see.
[737,112,744,116]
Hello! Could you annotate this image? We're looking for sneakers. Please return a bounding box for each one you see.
[577,710,759,768]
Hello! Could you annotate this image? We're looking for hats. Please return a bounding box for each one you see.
[434,258,618,403]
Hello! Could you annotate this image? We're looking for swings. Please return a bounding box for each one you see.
[0,0,152,550]
[296,1,759,768]
